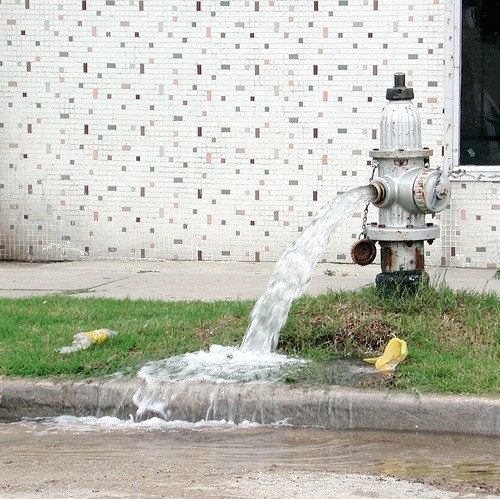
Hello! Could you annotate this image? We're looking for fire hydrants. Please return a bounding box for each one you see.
[351,72,452,298]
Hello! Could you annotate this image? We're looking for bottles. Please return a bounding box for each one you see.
[73,328,118,352]
